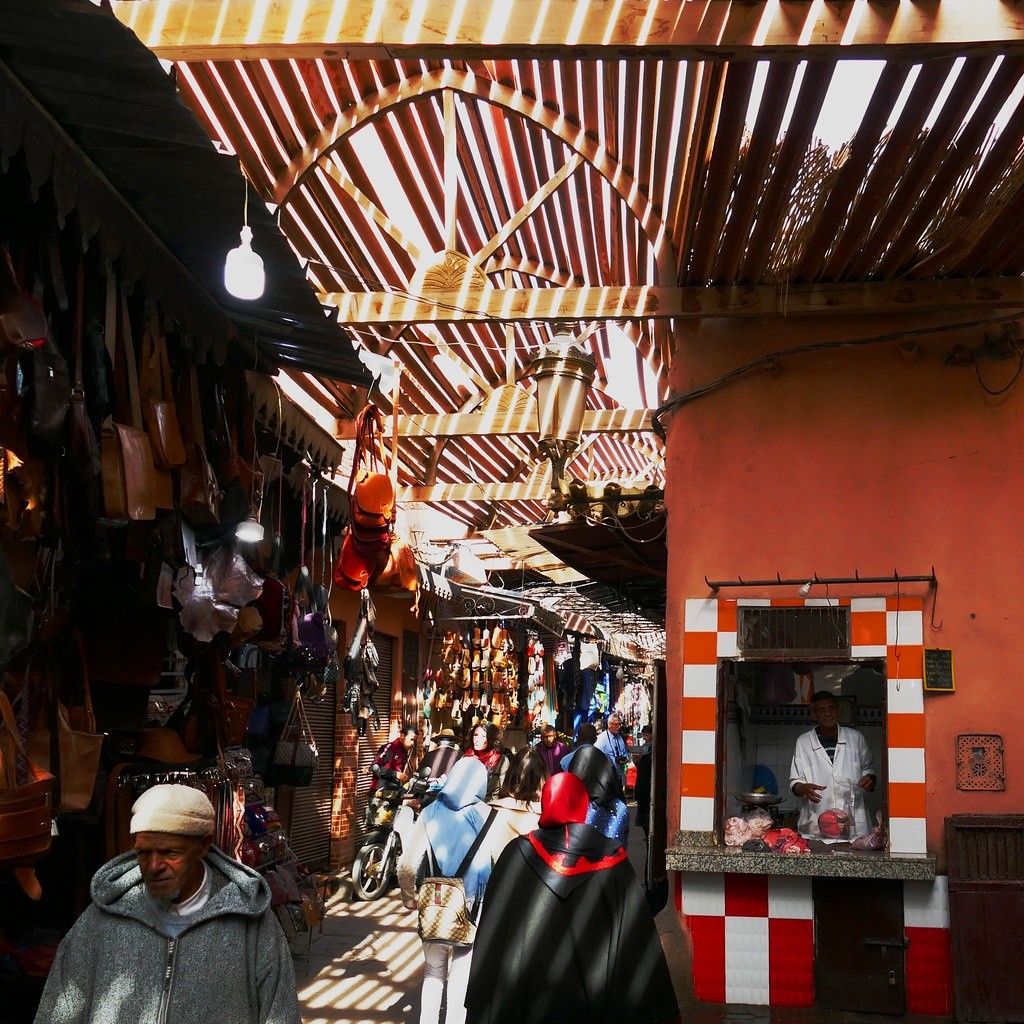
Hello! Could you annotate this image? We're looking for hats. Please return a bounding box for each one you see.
[130,784,215,836]
[642,726,652,733]
[430,729,458,742]
[811,690,833,704]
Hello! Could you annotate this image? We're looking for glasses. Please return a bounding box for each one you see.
[611,722,623,727]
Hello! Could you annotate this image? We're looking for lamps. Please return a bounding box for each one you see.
[798,577,816,597]
[529,327,667,544]
[235,491,265,543]
[223,180,266,299]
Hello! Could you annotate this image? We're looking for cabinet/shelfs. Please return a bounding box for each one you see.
[218,746,323,975]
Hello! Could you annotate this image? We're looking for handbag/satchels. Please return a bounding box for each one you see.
[0,243,396,946]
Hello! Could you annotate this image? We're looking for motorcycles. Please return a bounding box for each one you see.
[352,762,447,900]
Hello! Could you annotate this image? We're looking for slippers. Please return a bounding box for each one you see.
[440,626,519,747]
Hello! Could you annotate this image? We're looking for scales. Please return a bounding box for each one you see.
[734,794,788,831]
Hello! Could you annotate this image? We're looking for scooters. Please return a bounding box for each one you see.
[241,791,310,942]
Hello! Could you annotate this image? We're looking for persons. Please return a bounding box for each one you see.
[33,784,299,1024]
[569,747,631,852]
[789,690,877,837]
[525,713,652,841]
[363,726,514,888]
[397,757,506,1024]
[463,772,683,1024]
[488,747,548,843]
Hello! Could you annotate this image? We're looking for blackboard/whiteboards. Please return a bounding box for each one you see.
[921,646,957,693]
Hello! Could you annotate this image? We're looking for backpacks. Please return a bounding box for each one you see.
[416,806,498,948]
